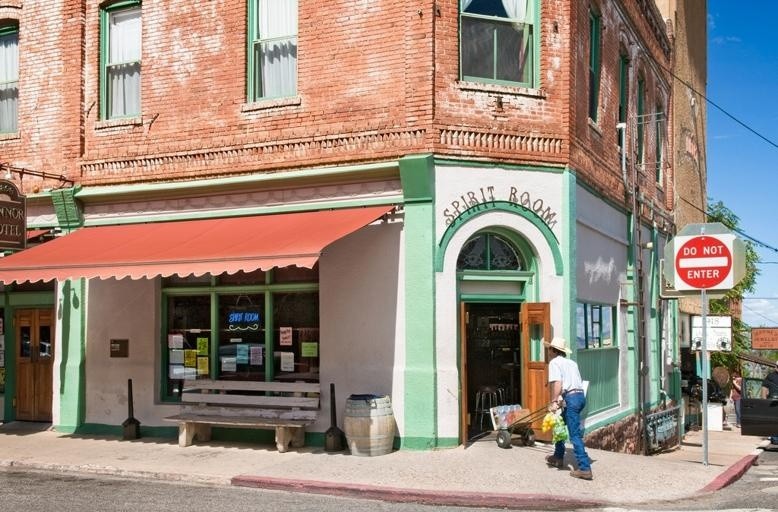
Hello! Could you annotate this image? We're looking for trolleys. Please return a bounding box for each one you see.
[488,400,563,448]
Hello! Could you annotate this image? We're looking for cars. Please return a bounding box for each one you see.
[740,376,777,437]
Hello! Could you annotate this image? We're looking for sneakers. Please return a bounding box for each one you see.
[544,454,564,469]
[569,469,593,480]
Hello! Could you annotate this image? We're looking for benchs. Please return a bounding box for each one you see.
[161,378,321,453]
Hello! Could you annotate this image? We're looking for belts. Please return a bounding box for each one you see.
[566,388,584,396]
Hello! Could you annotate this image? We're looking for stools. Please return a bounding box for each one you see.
[472,375,507,431]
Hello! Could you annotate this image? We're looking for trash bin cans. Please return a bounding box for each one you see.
[703,403,724,432]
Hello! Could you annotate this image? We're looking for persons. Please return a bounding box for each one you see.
[730,377,742,428]
[761,360,778,445]
[542,336,593,480]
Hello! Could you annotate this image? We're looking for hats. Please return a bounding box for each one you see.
[542,335,573,354]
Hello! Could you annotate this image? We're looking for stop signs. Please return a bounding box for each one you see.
[673,233,735,292]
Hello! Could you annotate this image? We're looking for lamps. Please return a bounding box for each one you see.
[0,162,16,181]
